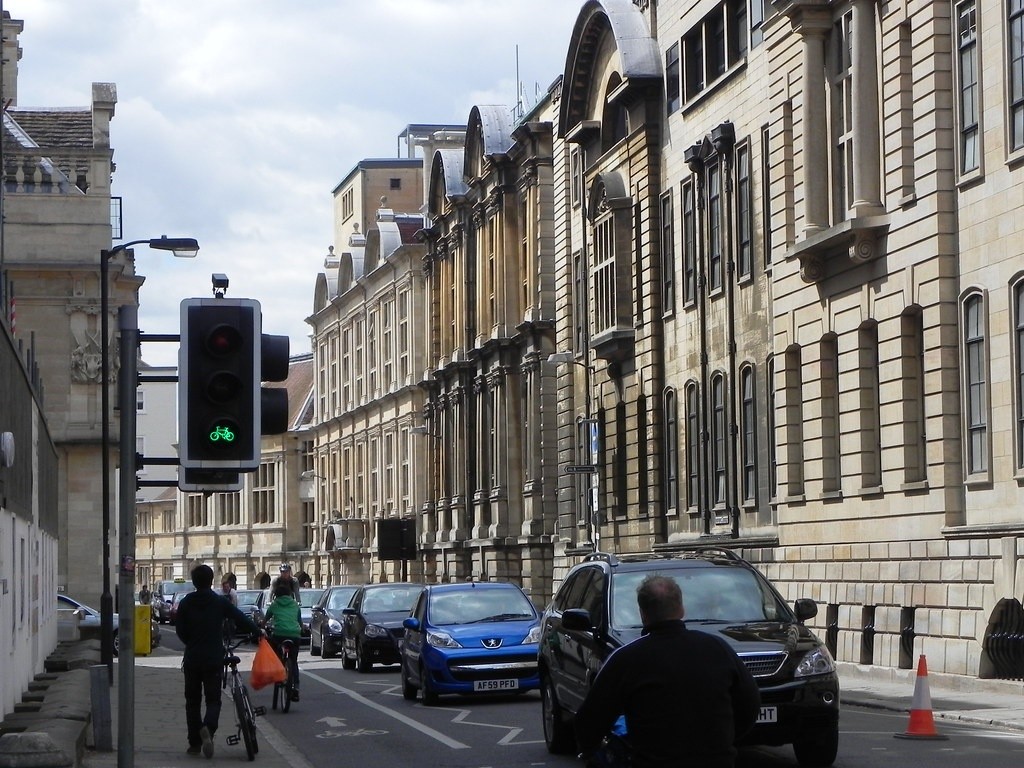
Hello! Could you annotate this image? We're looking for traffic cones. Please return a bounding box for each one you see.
[893,655,950,740]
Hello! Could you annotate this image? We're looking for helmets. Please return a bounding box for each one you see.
[279,564,292,572]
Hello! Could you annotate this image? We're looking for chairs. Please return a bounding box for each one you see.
[367,596,384,609]
[335,591,351,605]
[438,596,459,616]
[244,597,256,604]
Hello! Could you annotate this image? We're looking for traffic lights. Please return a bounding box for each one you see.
[180,298,262,472]
[261,332,290,435]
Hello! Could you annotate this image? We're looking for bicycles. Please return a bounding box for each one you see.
[258,625,305,714]
[224,634,268,761]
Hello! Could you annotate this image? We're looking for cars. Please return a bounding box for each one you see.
[236,589,261,623]
[309,585,362,659]
[340,582,429,674]
[401,579,543,708]
[57,594,162,658]
[294,587,327,640]
[250,589,274,638]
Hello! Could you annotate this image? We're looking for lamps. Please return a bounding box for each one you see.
[302,471,327,481]
[410,426,442,440]
[547,353,595,372]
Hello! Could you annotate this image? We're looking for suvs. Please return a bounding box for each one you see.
[537,545,842,768]
[150,577,197,626]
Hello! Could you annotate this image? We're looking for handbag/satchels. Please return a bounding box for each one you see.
[250,638,288,690]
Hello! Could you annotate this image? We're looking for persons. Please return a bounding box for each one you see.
[175,565,266,759]
[139,585,152,605]
[573,575,761,768]
[266,564,302,606]
[151,616,162,647]
[260,576,305,701]
[214,582,237,608]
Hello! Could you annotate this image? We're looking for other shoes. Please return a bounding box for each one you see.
[199,727,214,758]
[292,686,301,702]
[186,745,200,755]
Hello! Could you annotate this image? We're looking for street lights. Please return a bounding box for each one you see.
[99,234,201,689]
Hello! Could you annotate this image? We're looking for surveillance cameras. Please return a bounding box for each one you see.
[212,273,228,288]
